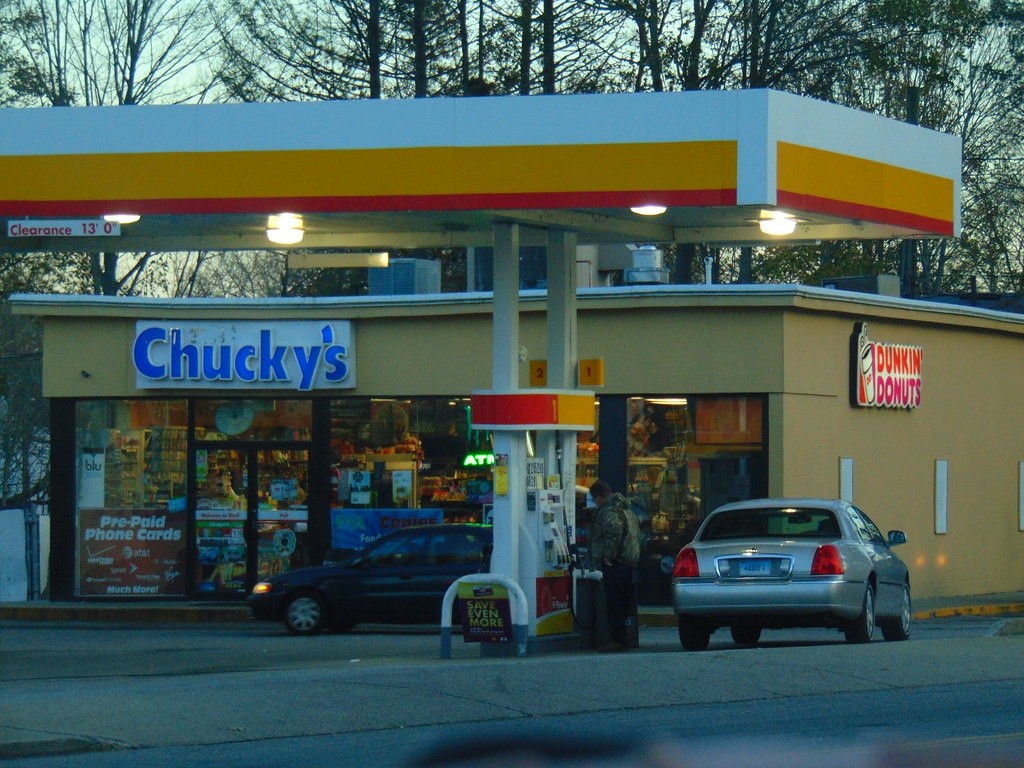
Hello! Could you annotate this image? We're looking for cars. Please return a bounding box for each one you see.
[249,522,494,636]
[671,496,912,645]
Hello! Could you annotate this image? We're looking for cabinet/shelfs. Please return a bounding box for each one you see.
[104,426,355,506]
[576,457,669,516]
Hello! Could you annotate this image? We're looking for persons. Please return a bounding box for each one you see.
[588,480,641,653]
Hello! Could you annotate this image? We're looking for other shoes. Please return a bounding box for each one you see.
[597,639,628,652]
